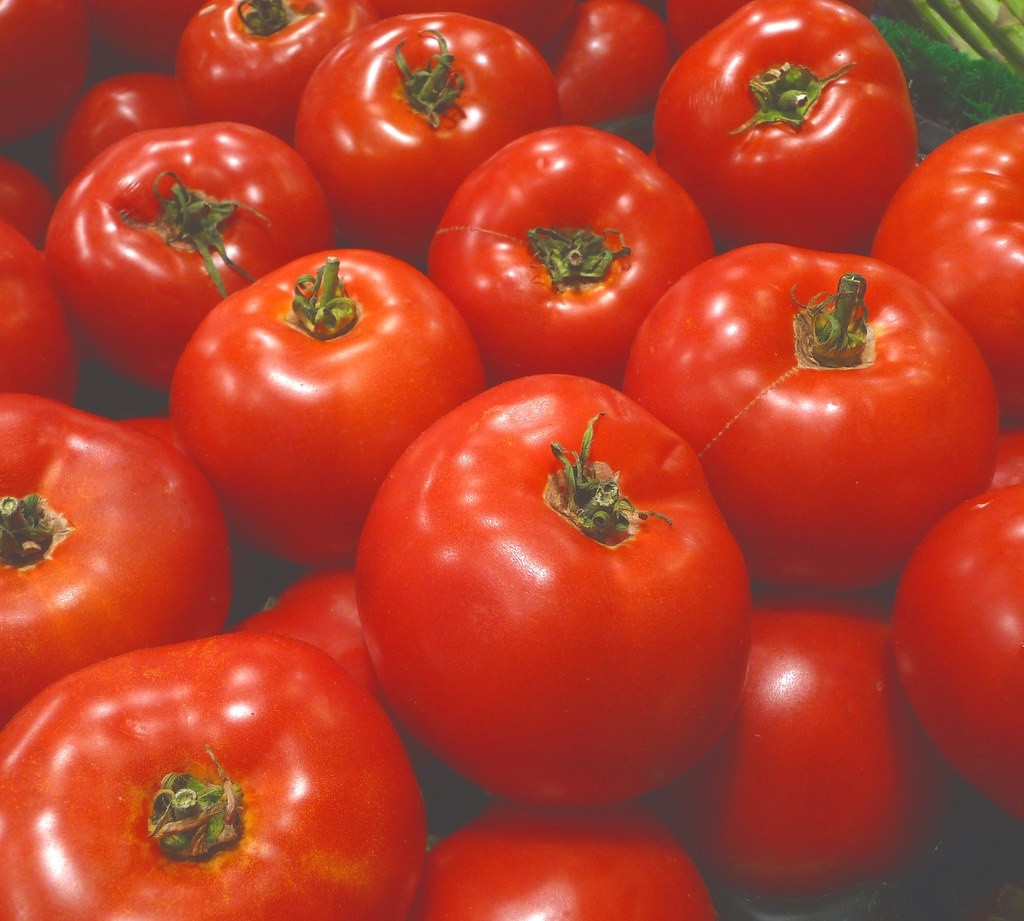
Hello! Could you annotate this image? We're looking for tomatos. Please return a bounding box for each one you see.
[0,0,1024,921]
